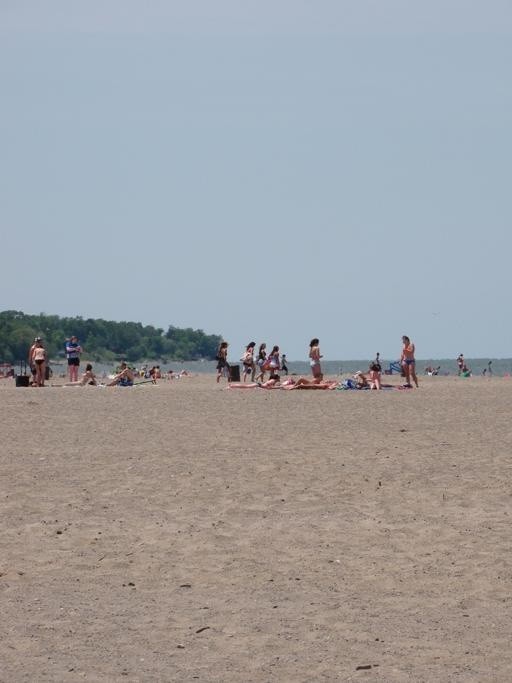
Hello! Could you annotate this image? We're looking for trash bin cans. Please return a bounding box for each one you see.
[229,365,240,382]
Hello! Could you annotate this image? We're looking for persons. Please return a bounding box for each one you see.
[0,335,188,386]
[215,336,493,390]
